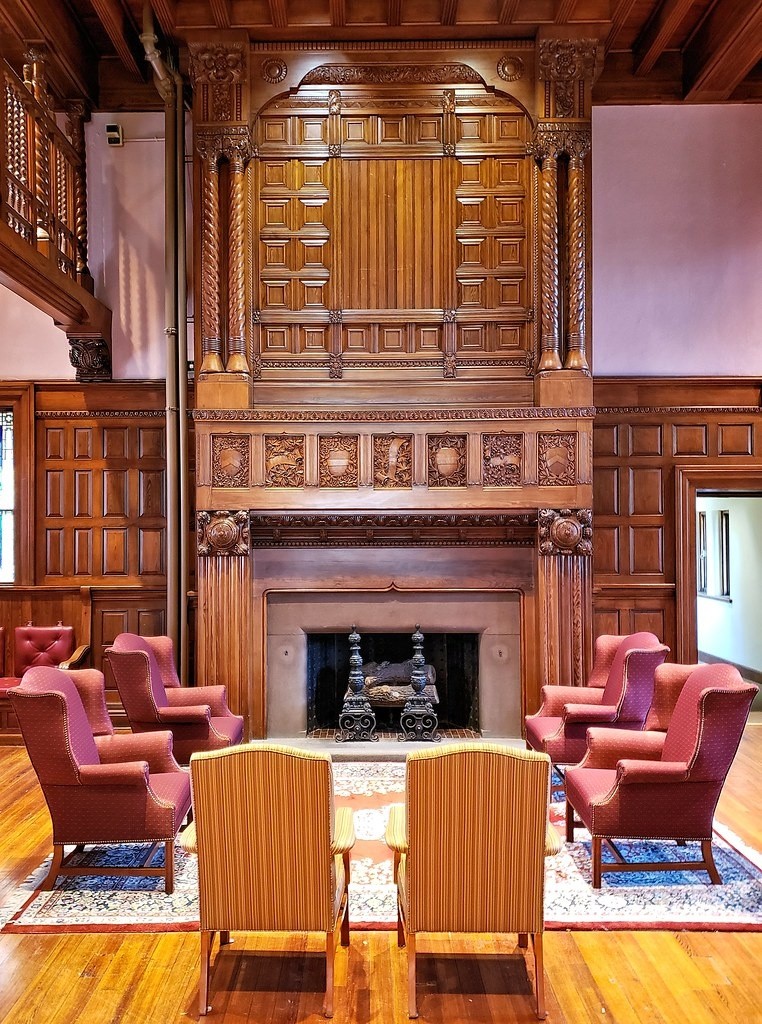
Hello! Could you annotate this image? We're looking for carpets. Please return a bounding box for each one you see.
[3,738,762,934]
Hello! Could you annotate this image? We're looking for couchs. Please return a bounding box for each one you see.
[104,633,243,764]
[526,631,673,764]
[5,665,192,894]
[565,662,760,887]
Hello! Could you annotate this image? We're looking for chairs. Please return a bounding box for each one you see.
[383,746,549,1023]
[181,741,354,1019]
[0,626,73,700]
